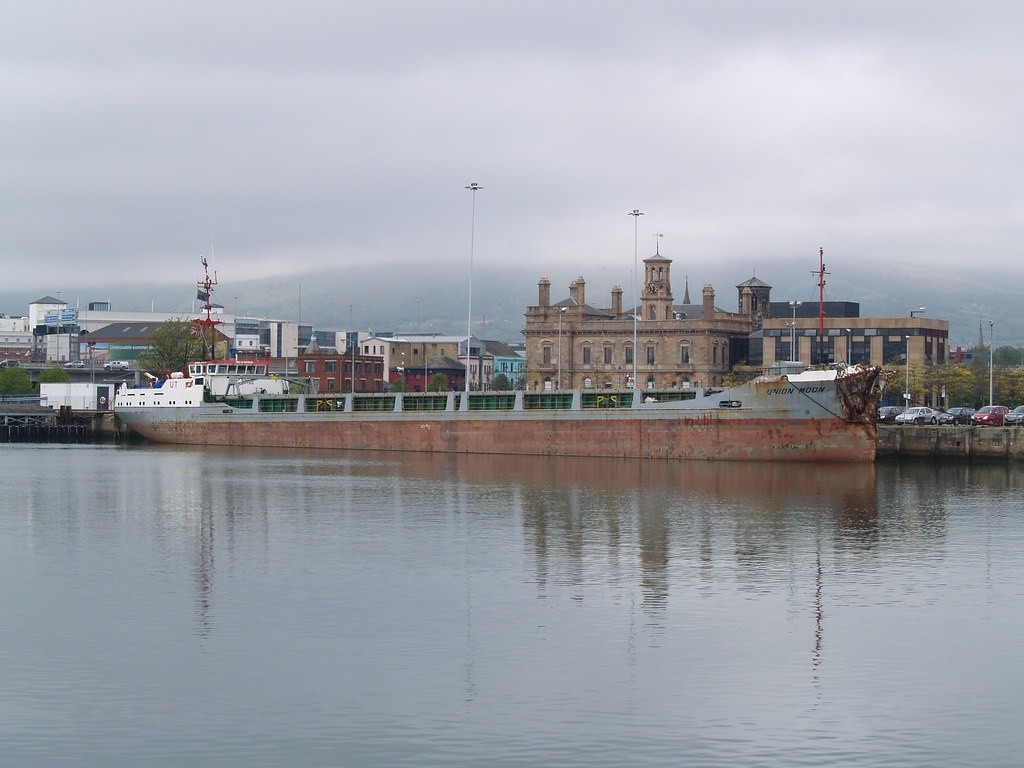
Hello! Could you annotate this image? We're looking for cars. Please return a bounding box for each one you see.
[1002,405,1024,427]
[894,406,936,425]
[971,406,1009,426]
[1,359,20,367]
[64,361,84,368]
[936,408,974,426]
[877,406,904,426]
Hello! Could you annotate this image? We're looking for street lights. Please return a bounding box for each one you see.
[464,183,484,392]
[846,328,851,365]
[417,300,420,333]
[905,335,911,410]
[56,291,61,365]
[789,300,802,359]
[628,209,644,388]
[553,307,567,390]
[785,322,795,361]
[84,305,87,366]
[988,320,993,406]
[341,338,354,393]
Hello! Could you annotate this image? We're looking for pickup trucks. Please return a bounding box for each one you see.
[103,362,129,371]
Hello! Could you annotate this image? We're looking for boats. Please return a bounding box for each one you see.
[116,255,882,465]
